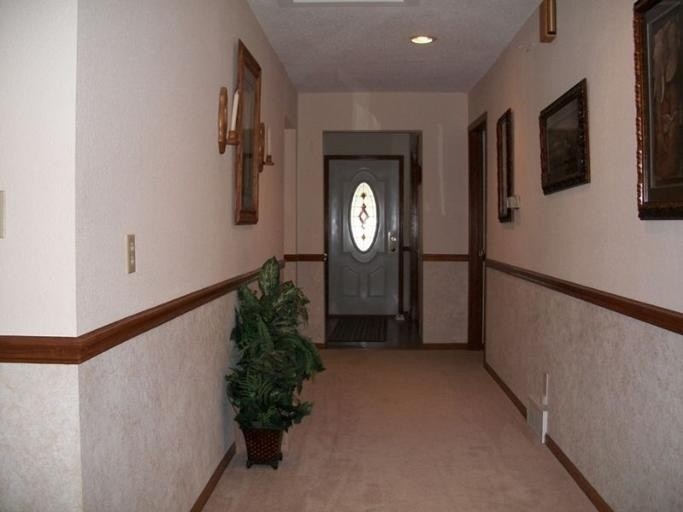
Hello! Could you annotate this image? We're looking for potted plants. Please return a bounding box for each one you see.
[227,260,325,469]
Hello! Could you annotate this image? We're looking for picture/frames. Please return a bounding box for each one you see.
[538,77,591,195]
[496,108,514,222]
[633,0,683,221]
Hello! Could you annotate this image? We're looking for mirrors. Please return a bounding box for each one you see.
[218,38,274,225]
